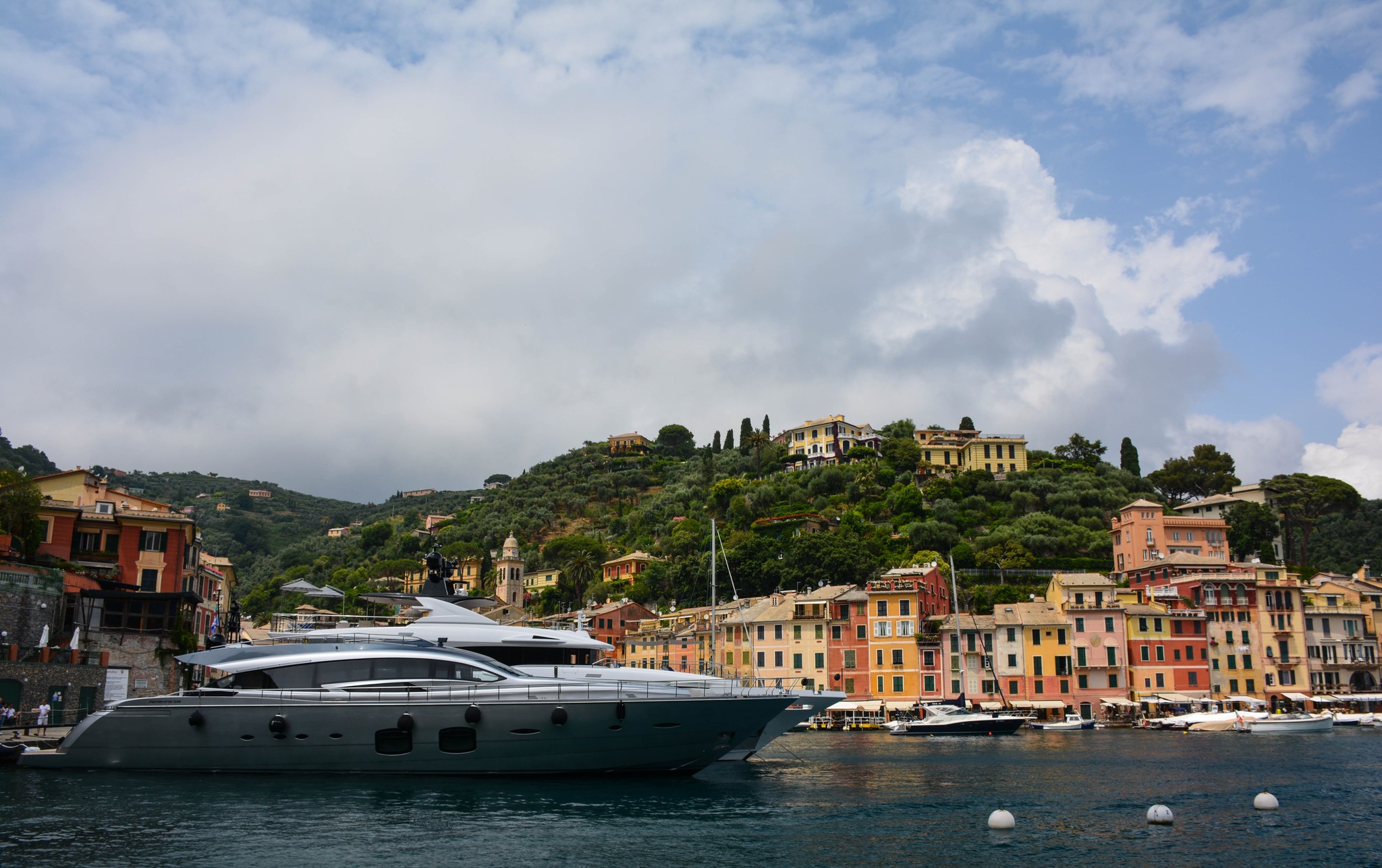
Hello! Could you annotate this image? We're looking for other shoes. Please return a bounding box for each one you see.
[0,733,3,735]
[33,733,39,736]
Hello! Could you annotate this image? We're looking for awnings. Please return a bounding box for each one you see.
[827,692,1382,711]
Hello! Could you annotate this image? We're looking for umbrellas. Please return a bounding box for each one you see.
[39,624,50,647]
[303,587,346,598]
[279,580,323,593]
[70,627,80,649]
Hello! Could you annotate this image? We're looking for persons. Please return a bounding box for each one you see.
[34,699,50,736]
[6,703,21,727]
[0,701,6,735]
[815,705,1302,737]
[0,697,3,721]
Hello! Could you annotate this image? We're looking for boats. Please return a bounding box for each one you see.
[1028,709,1381,733]
[17,516,850,775]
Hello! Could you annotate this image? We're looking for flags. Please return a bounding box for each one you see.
[211,614,219,633]
[213,601,218,615]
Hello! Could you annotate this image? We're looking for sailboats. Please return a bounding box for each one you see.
[889,550,1039,737]
